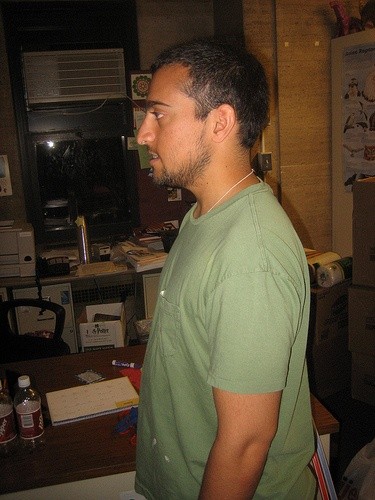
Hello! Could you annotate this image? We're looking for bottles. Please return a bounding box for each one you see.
[0,379,19,457]
[13,374,47,452]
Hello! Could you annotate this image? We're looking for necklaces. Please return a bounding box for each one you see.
[206,169,256,213]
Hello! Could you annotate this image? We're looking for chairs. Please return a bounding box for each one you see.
[0,298,70,365]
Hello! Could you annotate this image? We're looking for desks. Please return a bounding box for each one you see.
[0,343,340,493]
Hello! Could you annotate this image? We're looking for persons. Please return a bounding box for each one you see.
[135,37,339,500]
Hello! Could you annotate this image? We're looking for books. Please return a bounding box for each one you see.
[46,375,139,425]
[122,247,168,272]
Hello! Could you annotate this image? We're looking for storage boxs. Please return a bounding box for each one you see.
[80,302,130,353]
[306,177,375,407]
